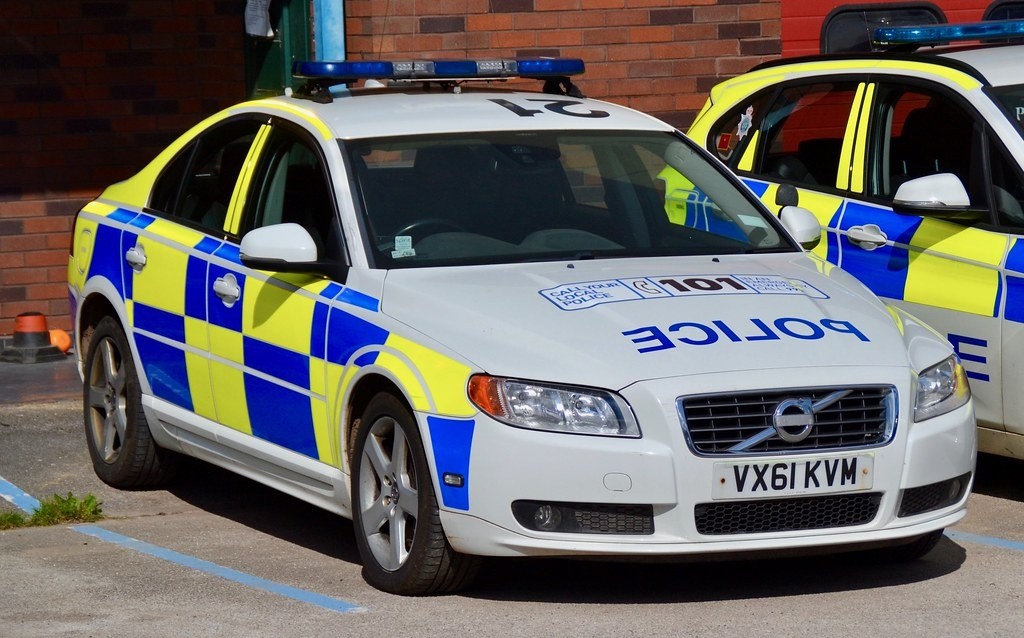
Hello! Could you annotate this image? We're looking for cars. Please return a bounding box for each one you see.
[66,65,974,596]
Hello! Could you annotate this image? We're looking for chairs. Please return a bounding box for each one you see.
[468,162,581,240]
[898,105,980,217]
[368,174,474,249]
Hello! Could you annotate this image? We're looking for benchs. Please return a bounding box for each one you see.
[770,138,898,203]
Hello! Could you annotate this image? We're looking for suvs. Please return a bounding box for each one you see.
[653,26,1023,462]
